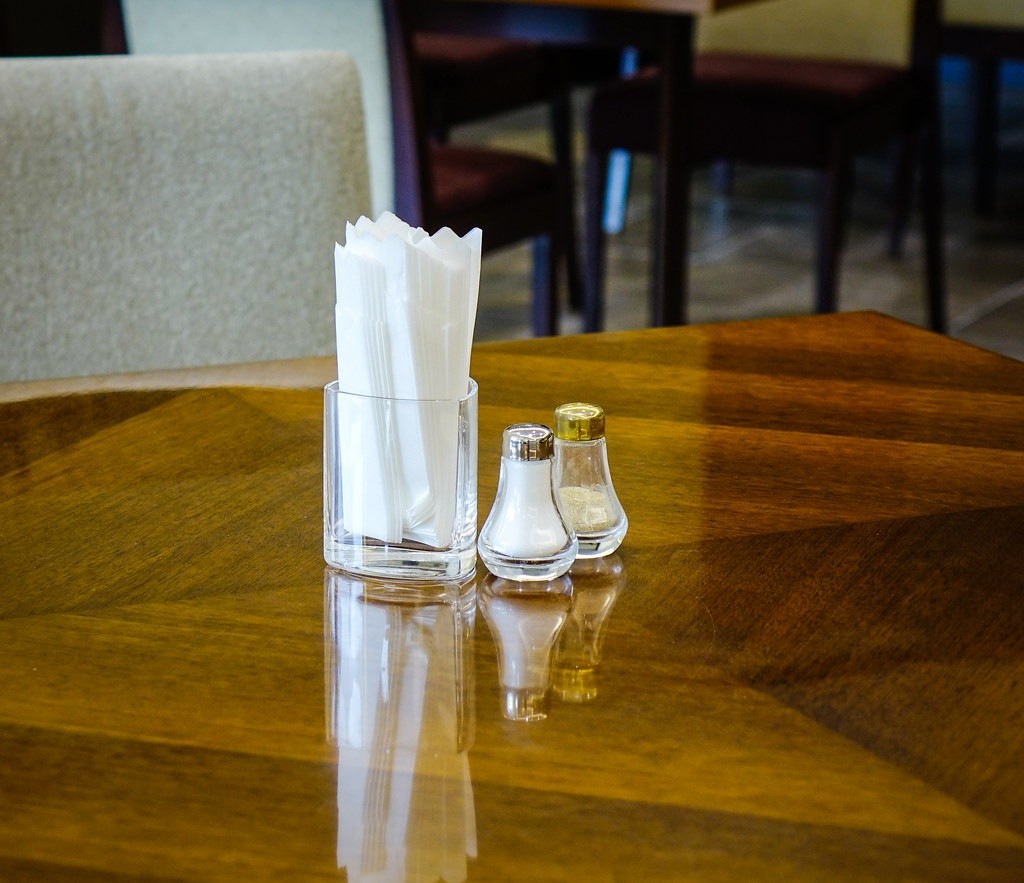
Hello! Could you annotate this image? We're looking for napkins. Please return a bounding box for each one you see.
[328,596,477,883]
[332,211,483,543]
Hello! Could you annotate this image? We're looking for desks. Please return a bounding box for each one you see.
[0,310,1024,883]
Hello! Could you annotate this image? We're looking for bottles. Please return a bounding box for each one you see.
[479,574,575,723]
[551,402,629,561]
[477,423,580,583]
[551,554,626,706]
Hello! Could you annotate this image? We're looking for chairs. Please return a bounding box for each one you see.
[101,1,1024,335]
[1,54,374,381]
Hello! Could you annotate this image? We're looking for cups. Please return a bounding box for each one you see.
[323,379,479,583]
[320,564,475,745]
